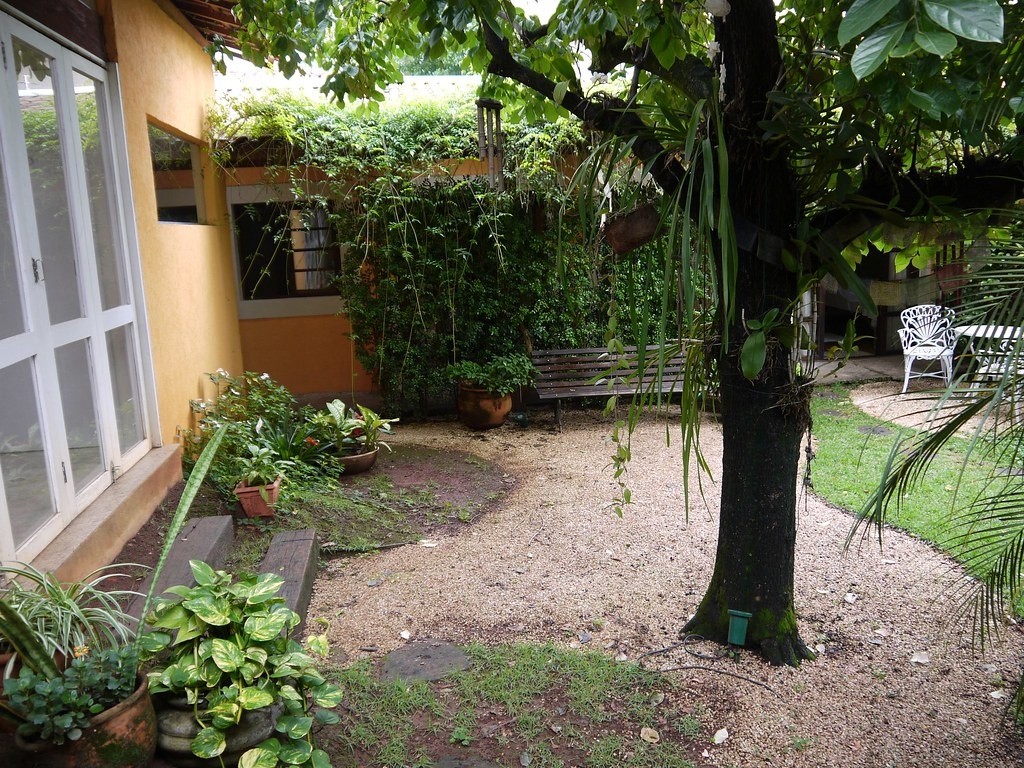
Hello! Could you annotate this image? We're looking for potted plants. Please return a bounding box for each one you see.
[2,643,159,768]
[146,560,344,768]
[234,444,295,518]
[446,353,545,428]
[303,399,400,473]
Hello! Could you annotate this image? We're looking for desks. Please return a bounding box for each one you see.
[955,325,1024,403]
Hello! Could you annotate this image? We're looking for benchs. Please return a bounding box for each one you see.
[531,343,722,432]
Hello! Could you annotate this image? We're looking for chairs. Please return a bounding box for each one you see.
[897,305,961,398]
[972,339,1024,428]
